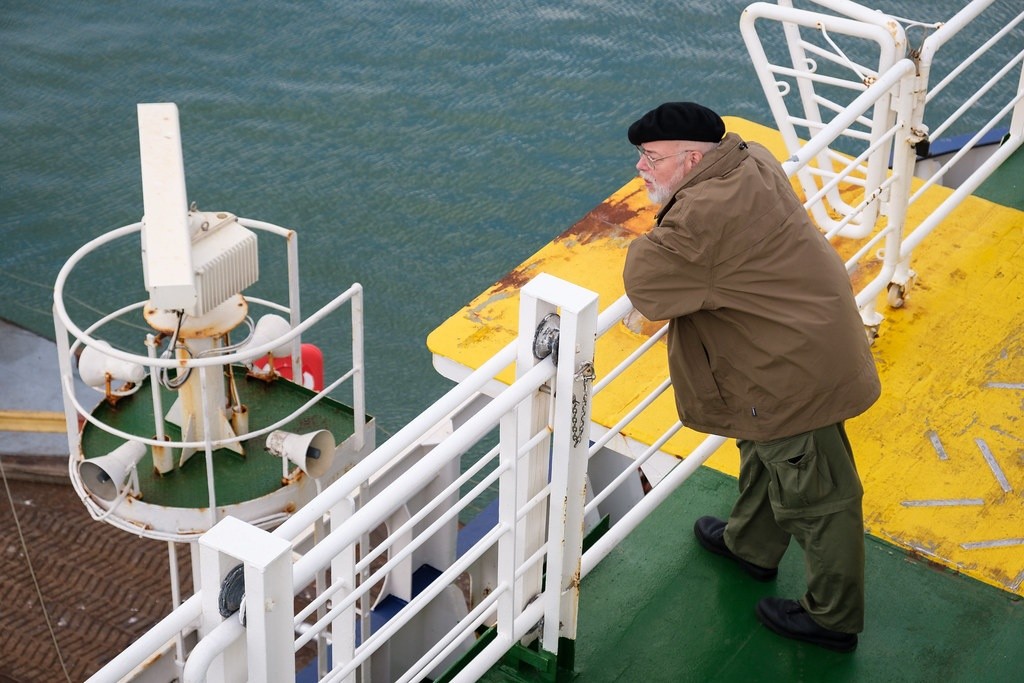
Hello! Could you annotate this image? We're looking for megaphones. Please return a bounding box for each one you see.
[263,430,336,476]
[236,314,292,364]
[77,441,147,503]
[78,340,144,388]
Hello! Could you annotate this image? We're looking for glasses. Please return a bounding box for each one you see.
[635,146,695,171]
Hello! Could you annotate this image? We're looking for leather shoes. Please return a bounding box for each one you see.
[757,596,858,653]
[694,516,778,583]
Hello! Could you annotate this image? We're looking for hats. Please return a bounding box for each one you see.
[628,102,725,146]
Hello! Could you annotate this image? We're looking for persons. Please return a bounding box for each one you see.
[623,102,883,655]
